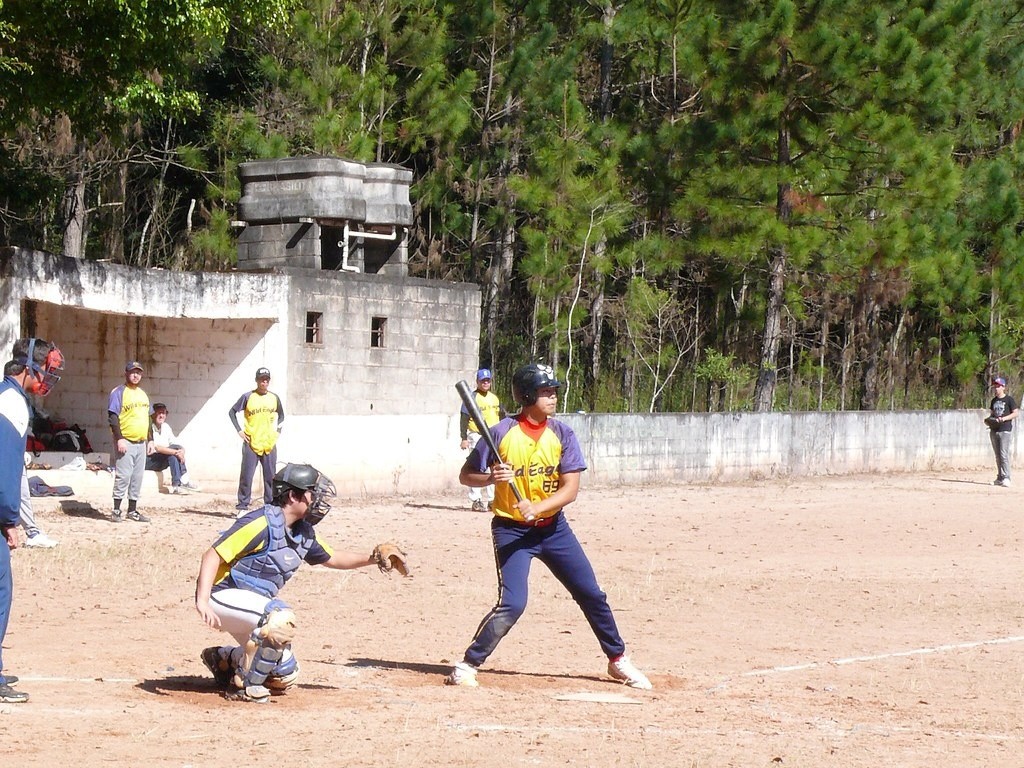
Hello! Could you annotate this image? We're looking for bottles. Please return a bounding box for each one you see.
[97,455,101,463]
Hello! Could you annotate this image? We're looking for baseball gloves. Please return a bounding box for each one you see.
[371,542,414,578]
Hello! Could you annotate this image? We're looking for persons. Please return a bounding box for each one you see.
[984,377,1019,487]
[0,337,66,705]
[20,451,58,548]
[145,403,198,495]
[460,368,504,512]
[228,367,285,520]
[443,363,652,690]
[107,362,154,523]
[194,462,411,704]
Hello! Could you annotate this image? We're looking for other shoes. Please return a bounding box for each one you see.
[25,531,58,547]
[488,501,494,509]
[172,479,204,495]
[1001,478,1012,486]
[472,498,488,512]
[989,479,1002,485]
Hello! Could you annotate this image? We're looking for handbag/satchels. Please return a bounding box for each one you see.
[26,405,93,457]
[27,476,74,496]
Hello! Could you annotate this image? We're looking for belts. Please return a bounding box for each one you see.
[495,514,555,529]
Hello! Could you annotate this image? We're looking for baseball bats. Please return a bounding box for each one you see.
[454,377,534,526]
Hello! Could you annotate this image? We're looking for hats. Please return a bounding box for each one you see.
[992,377,1006,386]
[126,361,144,372]
[477,369,490,381]
[153,403,167,410]
[256,367,270,380]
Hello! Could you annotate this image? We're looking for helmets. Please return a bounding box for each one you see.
[271,461,337,526]
[513,364,561,406]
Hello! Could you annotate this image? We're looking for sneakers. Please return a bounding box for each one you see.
[126,510,150,521]
[0,683,30,702]
[200,646,269,703]
[59,456,86,470]
[111,509,123,522]
[448,660,478,688]
[607,654,653,689]
[3,675,19,686]
